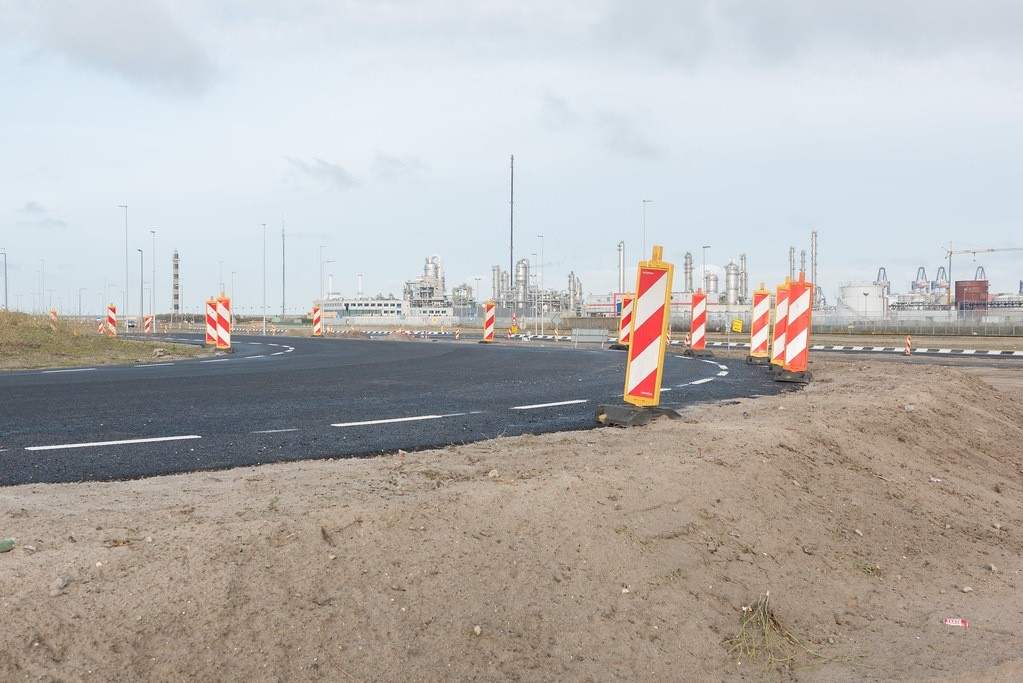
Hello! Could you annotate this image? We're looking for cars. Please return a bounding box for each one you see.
[128,320,135,327]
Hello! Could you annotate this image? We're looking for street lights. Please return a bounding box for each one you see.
[531,253,541,317]
[703,246,712,292]
[118,205,130,332]
[150,230,156,332]
[137,248,147,332]
[476,278,481,317]
[537,235,546,338]
[262,223,272,335]
[642,198,654,259]
[318,243,334,334]
